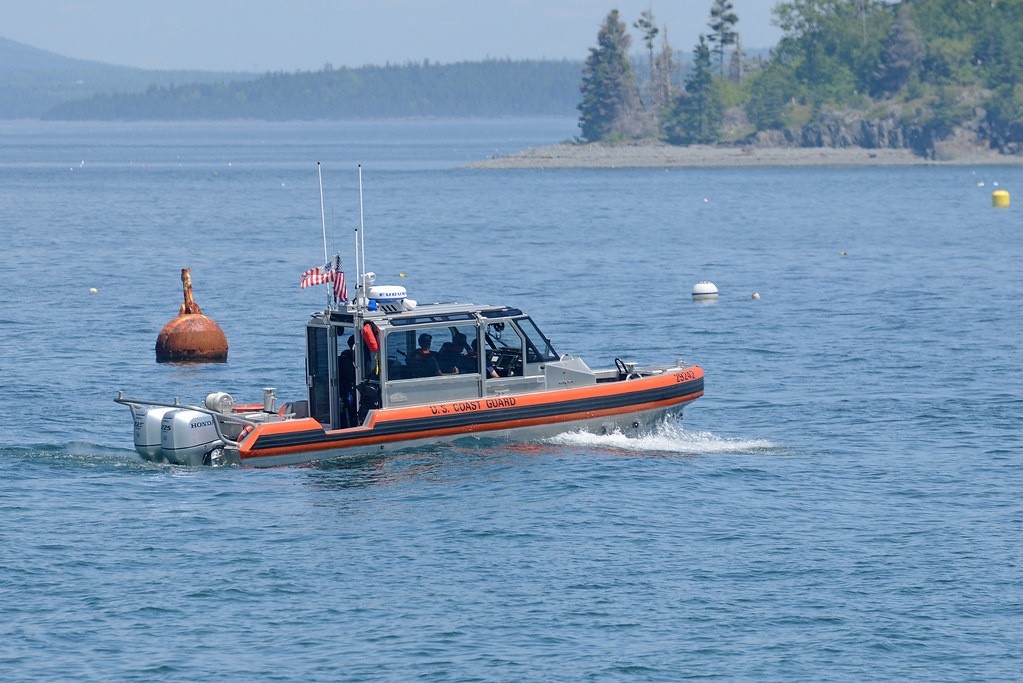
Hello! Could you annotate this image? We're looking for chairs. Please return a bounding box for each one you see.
[383,348,477,380]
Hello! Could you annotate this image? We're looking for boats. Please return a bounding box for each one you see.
[108,157,709,467]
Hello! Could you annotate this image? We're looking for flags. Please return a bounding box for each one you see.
[300,254,335,289]
[333,256,348,307]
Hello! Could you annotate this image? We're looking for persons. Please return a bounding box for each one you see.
[338,335,356,428]
[405,333,501,378]
